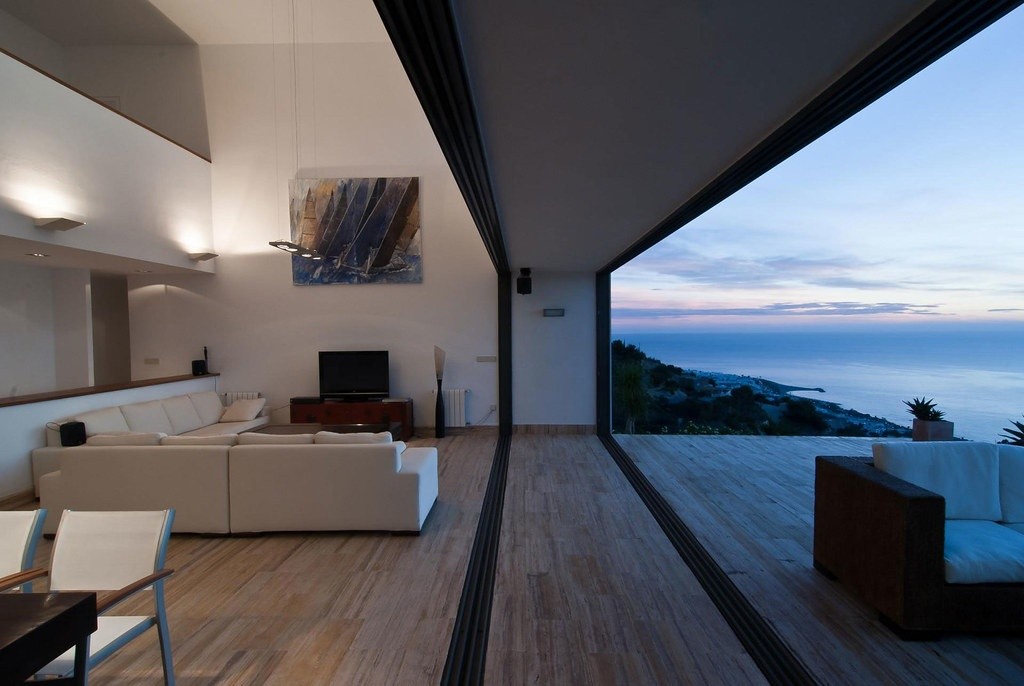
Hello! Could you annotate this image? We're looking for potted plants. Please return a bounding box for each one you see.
[902,395,954,442]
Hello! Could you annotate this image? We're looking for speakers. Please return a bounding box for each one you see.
[192,360,205,376]
[517,277,532,294]
[61,422,86,446]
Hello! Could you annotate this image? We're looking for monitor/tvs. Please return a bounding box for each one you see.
[318,351,389,403]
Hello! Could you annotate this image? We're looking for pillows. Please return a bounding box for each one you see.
[870,442,1003,522]
[996,443,1024,523]
[220,397,265,421]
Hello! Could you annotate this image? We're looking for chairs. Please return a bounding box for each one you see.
[29,510,175,685]
[1,510,48,591]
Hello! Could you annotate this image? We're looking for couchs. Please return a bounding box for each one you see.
[811,443,1024,638]
[30,389,439,532]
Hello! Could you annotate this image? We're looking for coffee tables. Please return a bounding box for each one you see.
[315,421,405,443]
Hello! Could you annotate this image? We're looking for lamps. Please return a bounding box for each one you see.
[516,267,532,294]
[190,253,218,261]
[269,0,329,262]
[434,345,446,439]
[34,218,85,231]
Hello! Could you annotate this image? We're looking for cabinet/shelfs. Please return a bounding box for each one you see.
[288,396,413,442]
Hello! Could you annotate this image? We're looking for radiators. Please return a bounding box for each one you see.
[220,391,260,405]
[442,388,469,427]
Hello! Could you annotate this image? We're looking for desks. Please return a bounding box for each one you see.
[0,591,97,686]
